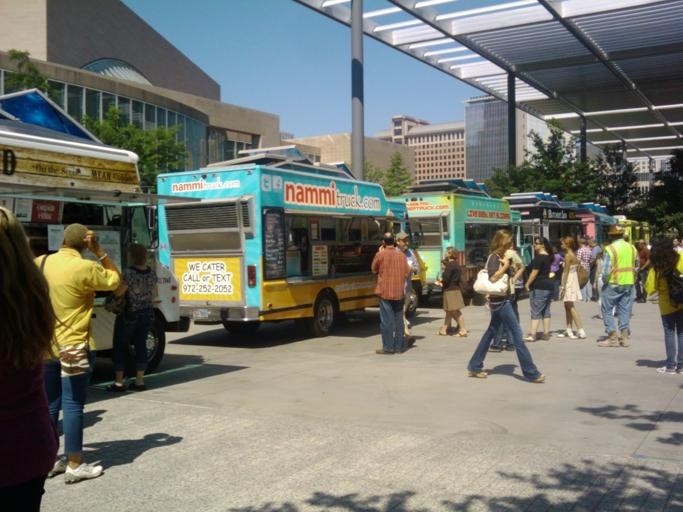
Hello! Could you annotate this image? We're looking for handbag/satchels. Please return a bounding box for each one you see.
[667,268,683,307]
[473,268,509,297]
[577,263,588,289]
[59,342,91,371]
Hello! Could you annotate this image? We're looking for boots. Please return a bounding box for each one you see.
[620,328,631,346]
[598,331,619,347]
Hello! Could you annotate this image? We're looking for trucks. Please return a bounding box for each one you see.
[396,173,533,304]
[501,188,654,262]
[154,143,430,339]
[0,87,182,380]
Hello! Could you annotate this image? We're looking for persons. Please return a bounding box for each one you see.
[393,232,419,332]
[106,243,157,391]
[644,237,682,375]
[29,223,124,485]
[1,205,58,512]
[465,226,545,384]
[371,232,411,354]
[431,225,683,354]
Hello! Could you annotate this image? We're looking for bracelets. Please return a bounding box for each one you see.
[98,252,106,261]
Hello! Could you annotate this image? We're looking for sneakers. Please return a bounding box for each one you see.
[466,369,488,378]
[48,456,67,478]
[558,329,574,339]
[657,366,678,375]
[528,375,545,384]
[65,462,103,483]
[571,332,586,340]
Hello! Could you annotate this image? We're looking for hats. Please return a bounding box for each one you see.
[396,232,408,240]
[608,224,625,235]
